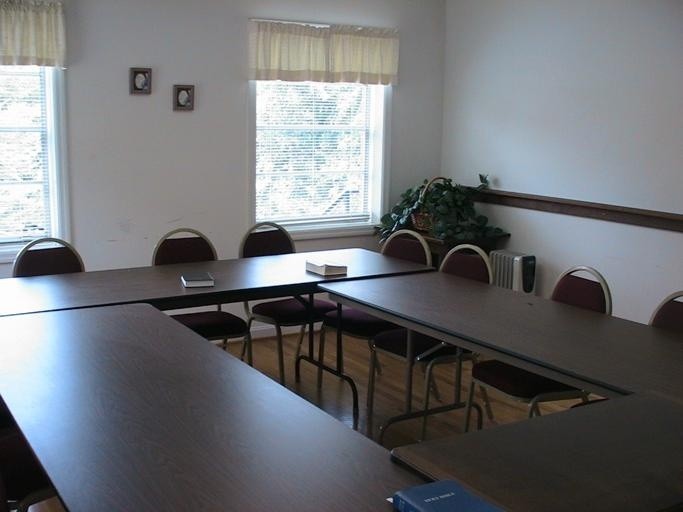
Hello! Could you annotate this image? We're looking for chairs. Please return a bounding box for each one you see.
[570,289,682,412]
[2,406,61,510]
[460,265,614,433]
[366,243,495,444]
[152,227,253,367]
[12,236,87,276]
[316,229,433,388]
[238,222,342,388]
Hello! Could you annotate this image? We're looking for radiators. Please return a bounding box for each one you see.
[488,248,537,297]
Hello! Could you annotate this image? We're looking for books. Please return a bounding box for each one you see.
[393,479,504,512]
[181,271,215,287]
[305,259,347,276]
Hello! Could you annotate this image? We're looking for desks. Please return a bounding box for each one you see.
[1,303,432,511]
[389,395,680,509]
[2,248,438,412]
[318,268,681,437]
[385,225,511,283]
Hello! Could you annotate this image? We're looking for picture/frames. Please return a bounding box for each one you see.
[171,83,197,113]
[128,65,155,97]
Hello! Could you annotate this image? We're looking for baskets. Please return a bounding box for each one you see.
[412,177,455,235]
